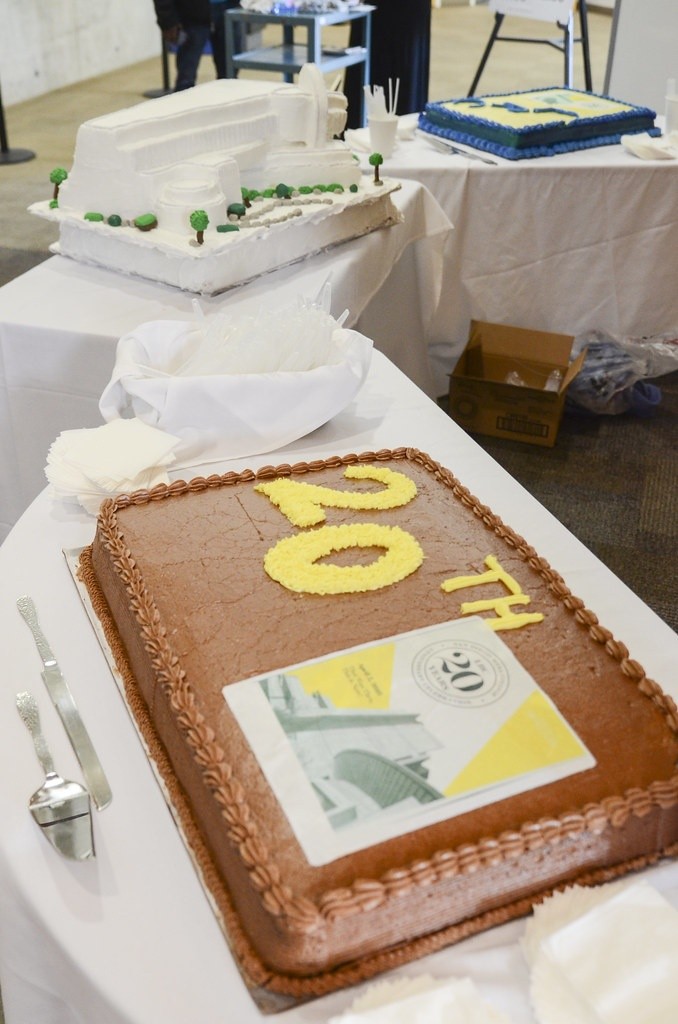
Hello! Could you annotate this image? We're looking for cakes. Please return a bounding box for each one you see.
[25,62,401,296]
[416,87,662,160]
[78,446,678,995]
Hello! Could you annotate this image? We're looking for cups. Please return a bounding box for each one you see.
[367,113,398,160]
[665,77,678,136]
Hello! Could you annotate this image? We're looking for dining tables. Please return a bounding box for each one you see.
[346,112,678,396]
[0,162,453,540]
[0,313,678,1024]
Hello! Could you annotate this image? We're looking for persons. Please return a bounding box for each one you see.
[141,0,247,99]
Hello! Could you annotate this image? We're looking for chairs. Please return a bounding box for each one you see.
[467,0,593,97]
[145,0,240,98]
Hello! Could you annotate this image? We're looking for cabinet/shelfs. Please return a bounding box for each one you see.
[225,6,378,135]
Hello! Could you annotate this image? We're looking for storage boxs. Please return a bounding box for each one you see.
[446,320,588,448]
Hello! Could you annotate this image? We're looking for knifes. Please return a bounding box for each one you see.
[19,597,113,811]
